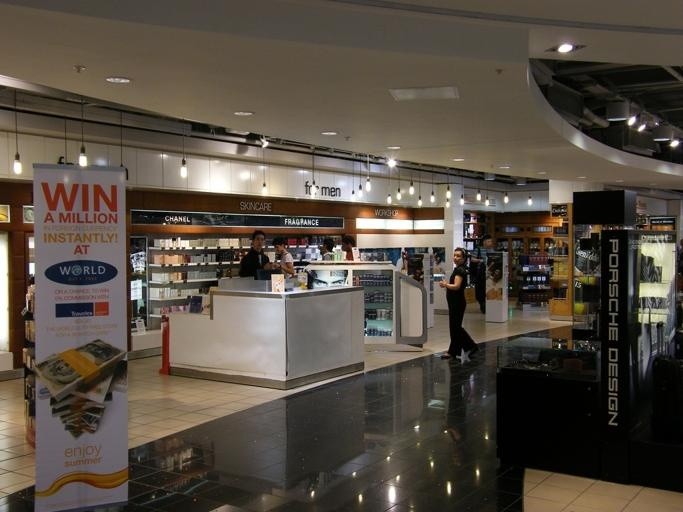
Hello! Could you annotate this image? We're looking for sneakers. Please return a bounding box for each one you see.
[441,353,456,359]
[468,348,478,357]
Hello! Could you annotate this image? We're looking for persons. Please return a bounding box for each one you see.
[271,236,294,279]
[441,362,473,467]
[396,250,407,271]
[439,247,481,360]
[341,235,356,260]
[487,262,502,289]
[409,269,423,283]
[239,231,274,280]
[471,234,496,314]
[320,237,335,260]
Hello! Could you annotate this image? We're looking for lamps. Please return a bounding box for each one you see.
[77,95,88,168]
[179,119,188,178]
[258,132,534,210]
[11,89,22,175]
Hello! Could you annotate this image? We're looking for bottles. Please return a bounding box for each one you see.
[545,243,567,255]
[530,241,538,251]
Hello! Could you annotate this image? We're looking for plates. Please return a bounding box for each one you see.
[44,352,95,383]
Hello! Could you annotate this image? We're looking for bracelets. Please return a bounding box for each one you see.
[445,283,448,288]
[278,263,281,268]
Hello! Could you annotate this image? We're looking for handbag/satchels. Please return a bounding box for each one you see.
[470,263,481,283]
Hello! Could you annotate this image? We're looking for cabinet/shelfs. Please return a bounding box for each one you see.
[149,236,340,324]
[350,270,395,343]
